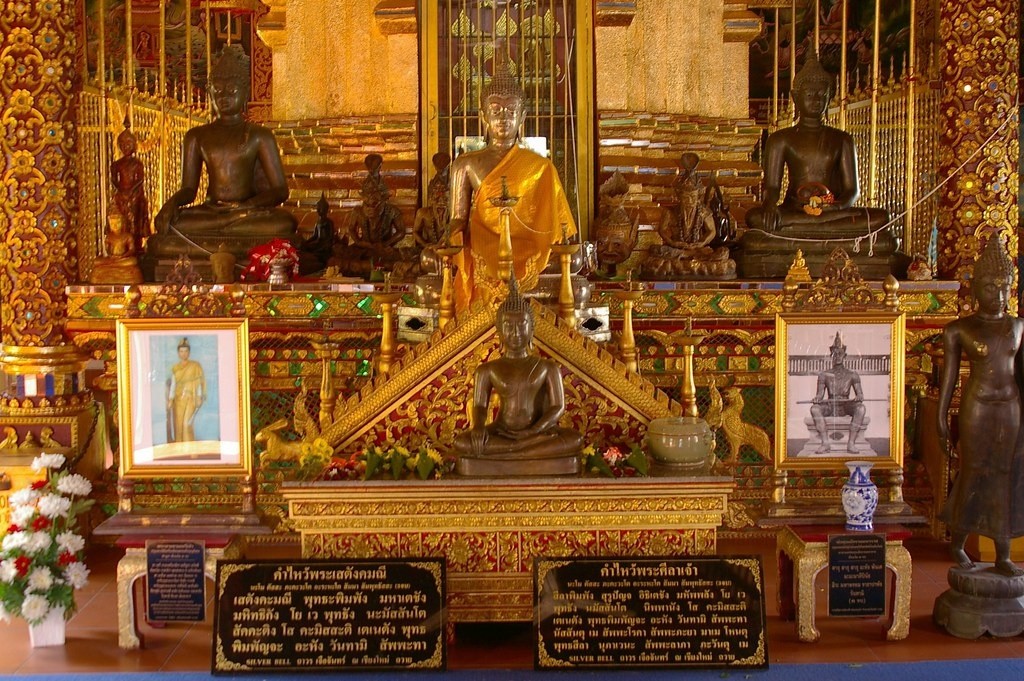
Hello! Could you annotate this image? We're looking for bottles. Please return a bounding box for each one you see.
[841,461,879,531]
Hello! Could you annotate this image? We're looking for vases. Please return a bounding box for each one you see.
[841,462,878,531]
[29,602,67,648]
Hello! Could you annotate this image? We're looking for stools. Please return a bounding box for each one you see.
[776,525,913,642]
[116,534,245,650]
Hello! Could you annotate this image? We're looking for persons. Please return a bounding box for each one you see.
[744,39,889,232]
[790,249,809,270]
[400,171,450,260]
[592,167,640,264]
[810,331,866,455]
[419,40,583,275]
[331,172,413,260]
[91,129,148,266]
[454,273,583,455]
[304,198,335,254]
[154,46,296,235]
[936,233,1024,572]
[649,170,729,261]
[167,337,206,441]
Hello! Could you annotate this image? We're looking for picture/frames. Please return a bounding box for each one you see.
[775,311,905,470]
[115,318,253,480]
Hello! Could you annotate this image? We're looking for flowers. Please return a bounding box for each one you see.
[582,439,652,478]
[0,451,96,625]
[298,439,460,480]
